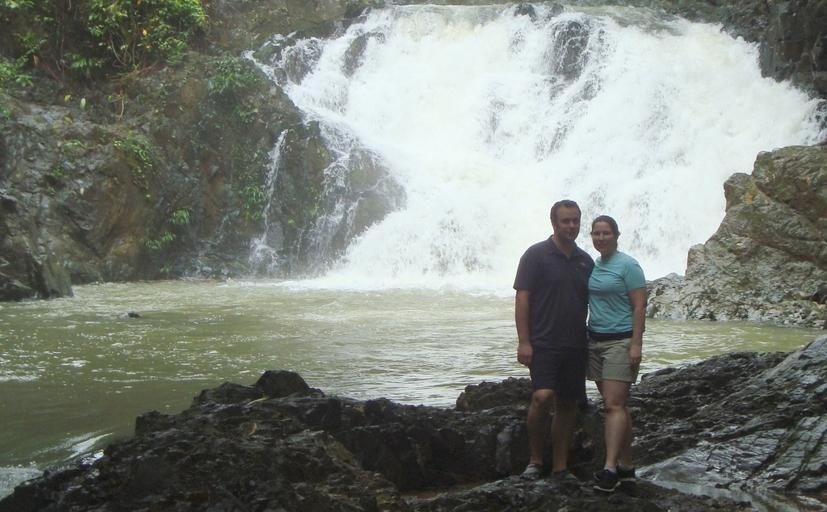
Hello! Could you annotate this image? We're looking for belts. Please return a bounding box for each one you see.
[588,331,634,340]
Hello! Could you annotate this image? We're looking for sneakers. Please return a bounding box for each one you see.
[520,463,578,482]
[593,468,636,492]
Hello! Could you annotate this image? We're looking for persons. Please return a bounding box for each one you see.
[513,200,647,492]
